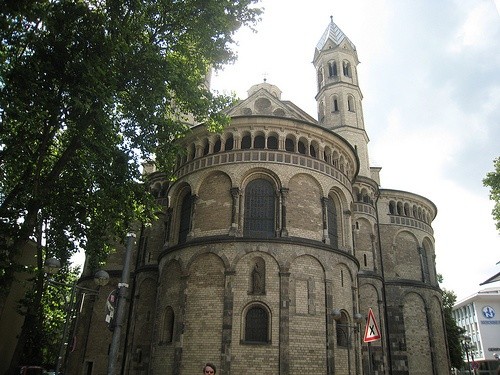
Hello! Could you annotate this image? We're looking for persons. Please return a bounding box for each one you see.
[202,362,216,375]
